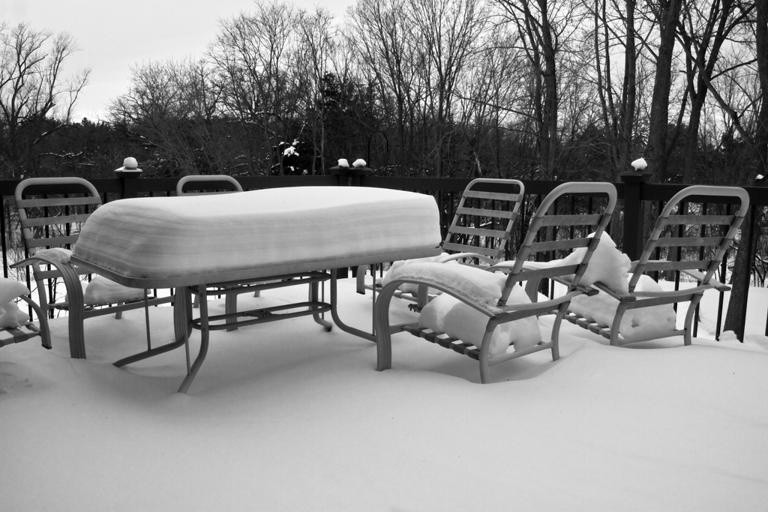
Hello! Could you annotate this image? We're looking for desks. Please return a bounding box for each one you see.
[70,185,443,394]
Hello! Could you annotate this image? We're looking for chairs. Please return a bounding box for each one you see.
[358,177,524,312]
[175,177,260,331]
[10,176,186,359]
[375,182,618,383]
[549,182,750,347]
